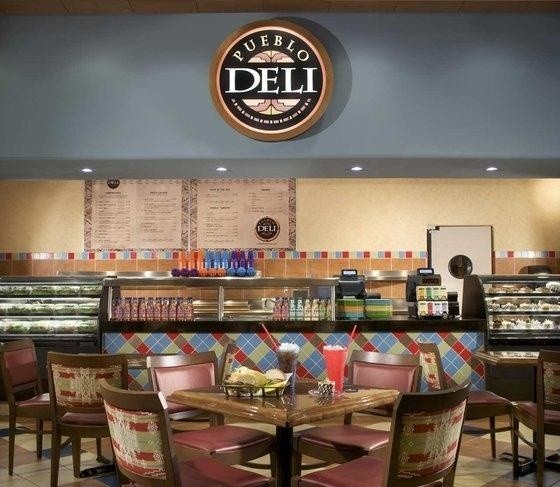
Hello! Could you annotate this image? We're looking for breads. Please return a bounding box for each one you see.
[229,366,286,392]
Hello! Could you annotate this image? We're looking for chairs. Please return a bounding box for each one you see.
[0,335,560,486]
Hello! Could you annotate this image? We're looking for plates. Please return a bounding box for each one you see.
[337,299,392,321]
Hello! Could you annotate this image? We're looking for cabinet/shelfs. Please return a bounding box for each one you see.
[0,275,119,389]
[462,272,560,402]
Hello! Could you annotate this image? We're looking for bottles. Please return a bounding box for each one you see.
[115,297,196,323]
[178,249,255,269]
[418,285,447,316]
[274,297,332,323]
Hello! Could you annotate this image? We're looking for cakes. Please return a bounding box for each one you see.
[486,281,560,329]
[0,285,102,334]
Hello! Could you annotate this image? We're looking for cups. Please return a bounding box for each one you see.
[276,348,298,393]
[323,348,348,394]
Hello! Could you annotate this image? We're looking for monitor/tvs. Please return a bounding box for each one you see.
[332,274,365,299]
[406,274,442,301]
